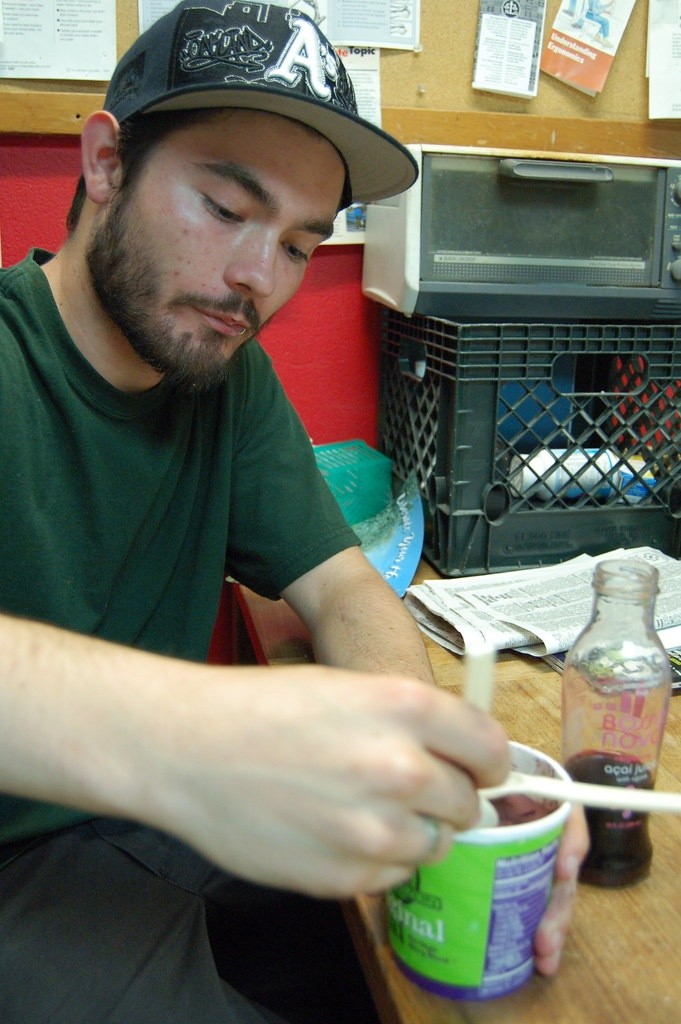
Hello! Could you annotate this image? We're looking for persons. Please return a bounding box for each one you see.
[1,0,590,1024]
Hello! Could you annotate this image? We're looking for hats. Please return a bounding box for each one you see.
[104,0,418,204]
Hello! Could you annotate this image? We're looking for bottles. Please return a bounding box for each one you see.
[553,556,673,885]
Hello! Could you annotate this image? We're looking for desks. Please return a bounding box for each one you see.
[230,551,681,1024]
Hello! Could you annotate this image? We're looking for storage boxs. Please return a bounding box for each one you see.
[312,440,391,528]
[380,316,680,573]
